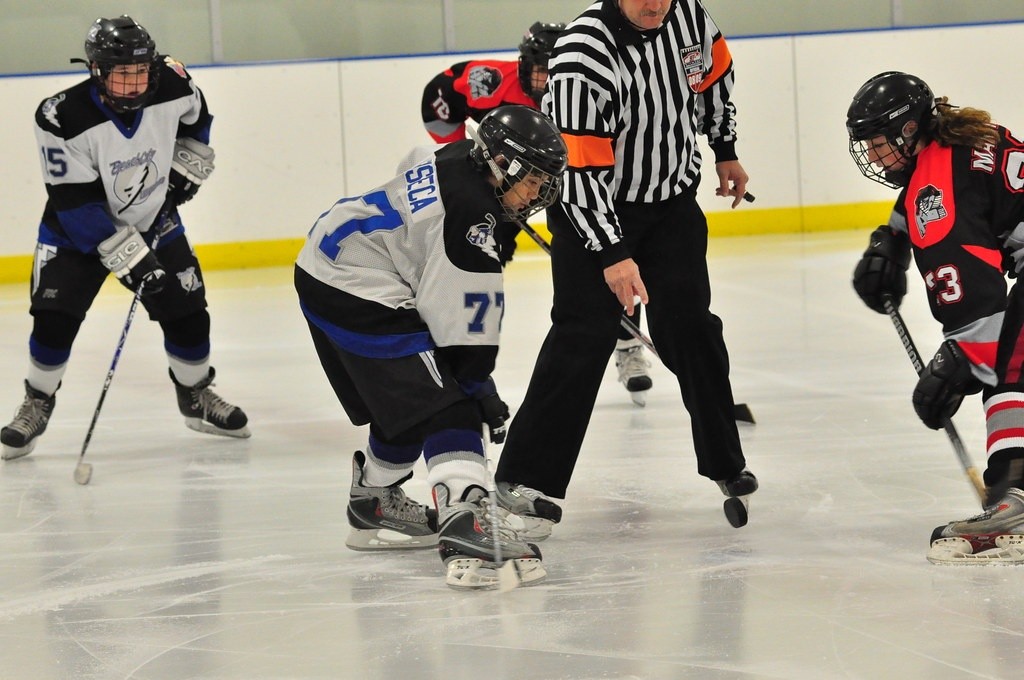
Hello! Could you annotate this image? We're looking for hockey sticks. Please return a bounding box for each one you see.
[481,419,547,590]
[883,295,987,503]
[73,200,174,486]
[515,217,756,427]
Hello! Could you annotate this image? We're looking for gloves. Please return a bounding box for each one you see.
[912,341,985,429]
[475,378,509,445]
[852,226,914,315]
[162,137,215,213]
[98,226,166,295]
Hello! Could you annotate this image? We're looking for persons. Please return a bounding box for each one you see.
[496,0,759,542]
[293,105,568,594]
[0,16,253,459]
[845,70,1023,569]
[424,20,654,409]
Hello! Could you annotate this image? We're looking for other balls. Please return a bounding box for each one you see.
[724,497,748,529]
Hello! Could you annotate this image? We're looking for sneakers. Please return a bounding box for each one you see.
[615,344,652,410]
[344,451,440,551]
[926,461,1024,567]
[430,480,547,589]
[0,379,62,461]
[713,471,760,528]
[169,365,250,438]
[494,480,562,526]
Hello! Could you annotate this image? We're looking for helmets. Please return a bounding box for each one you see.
[517,21,572,104]
[473,105,567,223]
[844,71,935,189]
[69,16,159,111]
